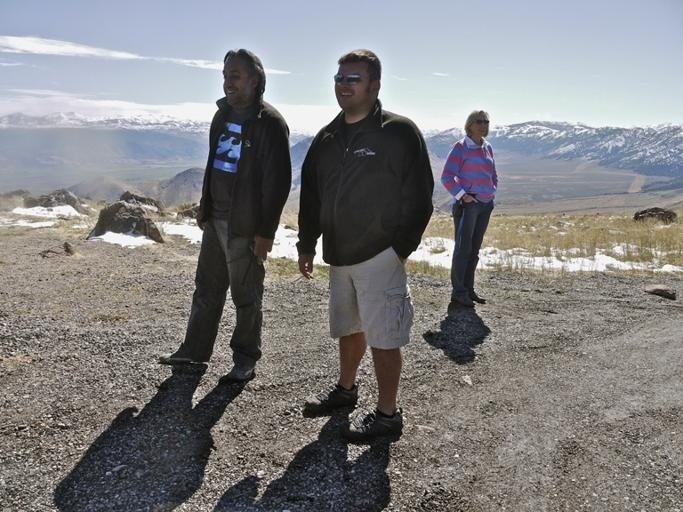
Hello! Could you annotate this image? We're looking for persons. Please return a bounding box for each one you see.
[441,110,497,306]
[158,49,292,380]
[296,48,434,441]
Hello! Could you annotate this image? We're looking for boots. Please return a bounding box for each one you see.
[450,288,487,307]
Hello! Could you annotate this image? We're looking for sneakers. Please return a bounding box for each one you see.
[229,364,255,380]
[159,342,210,363]
[305,383,358,410]
[341,406,403,440]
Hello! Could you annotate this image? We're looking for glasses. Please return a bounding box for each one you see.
[476,119,489,125]
[333,73,361,84]
